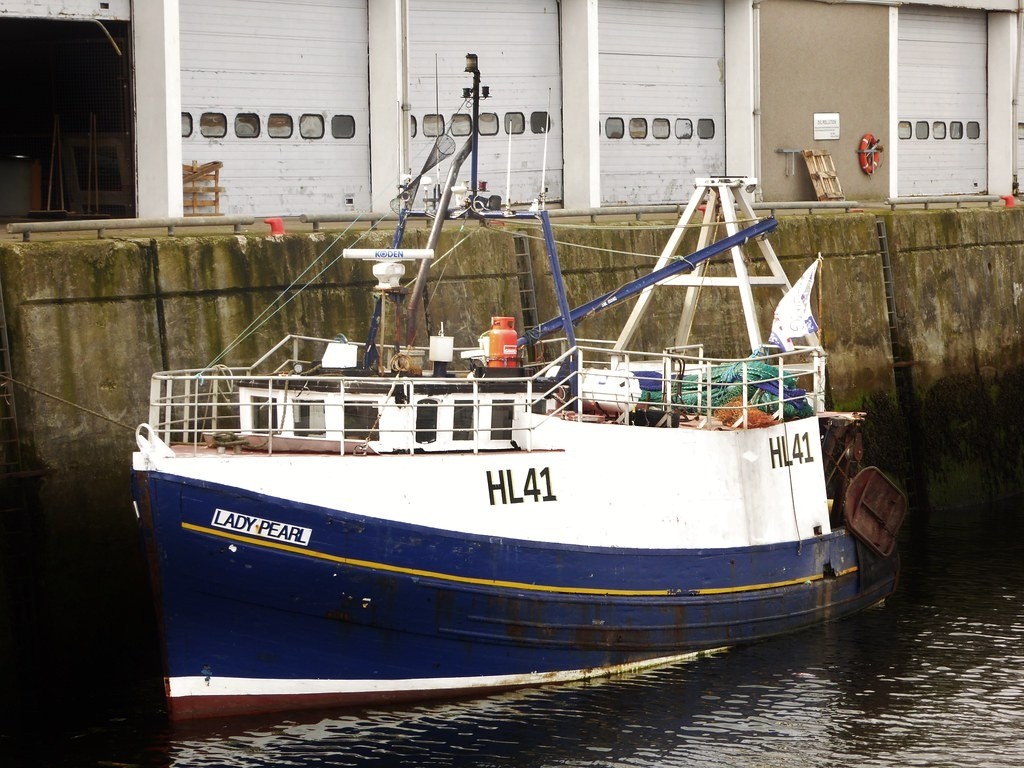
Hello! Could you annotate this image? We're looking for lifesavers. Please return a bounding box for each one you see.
[855,131,884,177]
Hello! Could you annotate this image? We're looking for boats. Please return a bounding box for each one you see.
[127,51,903,721]
